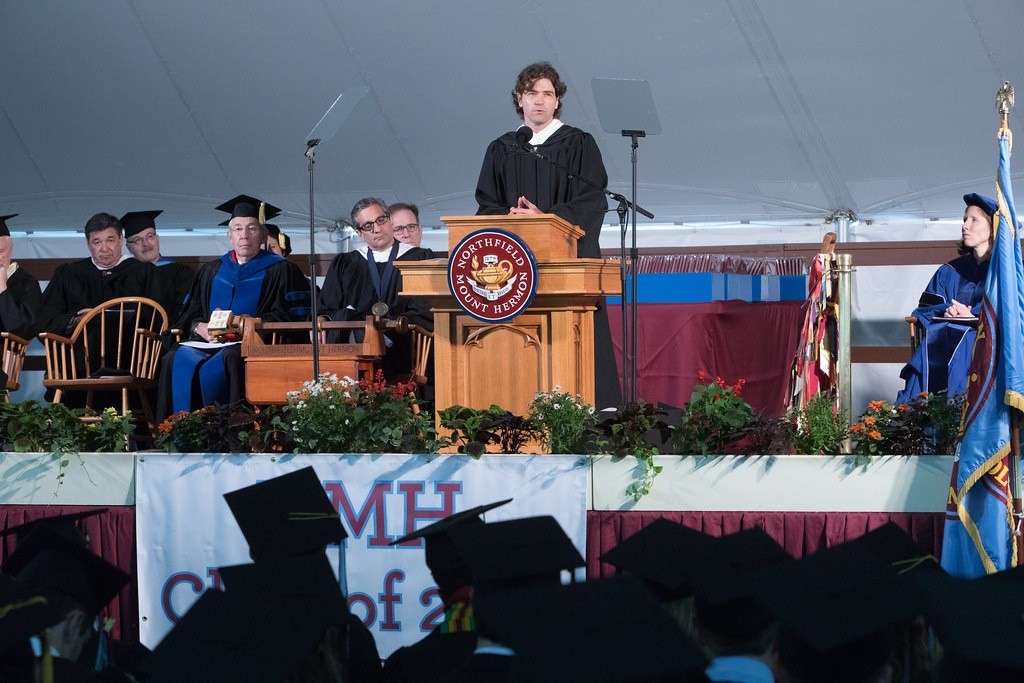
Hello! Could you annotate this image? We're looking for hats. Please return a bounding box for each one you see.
[215,195,282,226]
[14,470,1018,677]
[119,210,163,238]
[265,224,292,257]
[0,214,19,237]
[963,193,1000,216]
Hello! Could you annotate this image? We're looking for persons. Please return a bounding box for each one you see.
[307,197,434,381]
[0,212,44,394]
[36,212,168,409]
[387,203,423,247]
[0,465,1024,683]
[120,210,197,329]
[259,223,292,258]
[156,194,310,420]
[891,193,999,407]
[474,61,626,411]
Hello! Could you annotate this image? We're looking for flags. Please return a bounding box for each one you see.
[939,136,1024,575]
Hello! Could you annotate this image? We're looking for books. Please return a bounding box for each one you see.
[930,316,980,325]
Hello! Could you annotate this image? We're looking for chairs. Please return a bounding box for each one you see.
[0,294,434,452]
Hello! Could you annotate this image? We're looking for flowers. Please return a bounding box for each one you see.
[151,400,219,442]
[696,367,755,414]
[286,363,361,444]
[83,400,134,439]
[351,368,421,404]
[846,390,928,442]
[922,387,968,421]
[536,380,602,430]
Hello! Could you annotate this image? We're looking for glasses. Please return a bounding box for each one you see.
[125,233,156,244]
[393,223,419,236]
[357,215,389,231]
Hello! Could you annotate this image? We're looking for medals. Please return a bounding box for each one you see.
[372,301,389,316]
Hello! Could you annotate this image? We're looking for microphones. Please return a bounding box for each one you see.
[512,126,533,149]
